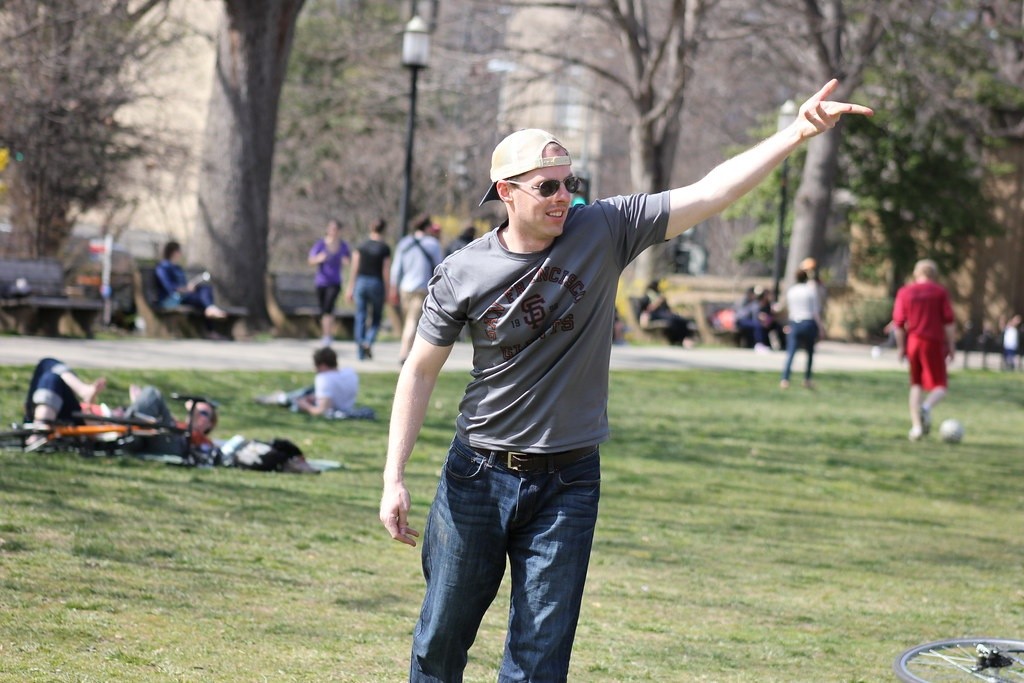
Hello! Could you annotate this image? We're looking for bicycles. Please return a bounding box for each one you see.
[0,390,221,469]
[893,637,1024,683]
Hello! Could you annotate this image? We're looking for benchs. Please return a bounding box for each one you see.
[1,256,103,337]
[137,262,250,341]
[626,298,674,342]
[699,301,748,348]
[265,269,354,339]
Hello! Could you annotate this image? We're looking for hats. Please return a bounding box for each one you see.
[477,129,573,207]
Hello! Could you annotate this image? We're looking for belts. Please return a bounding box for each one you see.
[471,443,598,472]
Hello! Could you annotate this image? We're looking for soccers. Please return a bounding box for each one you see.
[939,420,965,444]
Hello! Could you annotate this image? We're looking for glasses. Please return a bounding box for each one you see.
[198,409,214,423]
[505,177,583,197]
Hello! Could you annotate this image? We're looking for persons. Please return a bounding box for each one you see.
[445,227,476,258]
[389,213,442,365]
[24,358,218,447]
[346,217,392,360]
[154,242,226,318]
[733,287,786,350]
[309,218,351,348]
[640,282,697,349]
[892,259,957,440]
[291,348,359,416]
[1003,314,1024,369]
[780,271,820,389]
[378,79,874,683]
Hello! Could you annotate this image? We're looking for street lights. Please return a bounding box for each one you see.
[482,56,598,180]
[772,97,799,308]
[396,9,436,237]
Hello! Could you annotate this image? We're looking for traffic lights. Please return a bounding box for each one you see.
[570,174,592,209]
